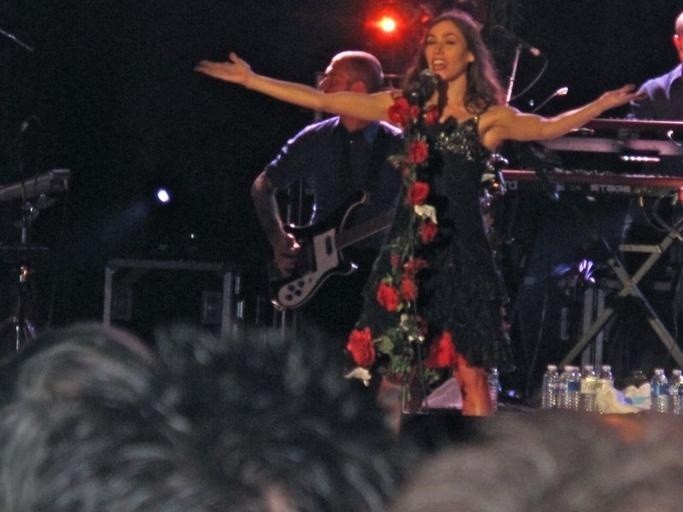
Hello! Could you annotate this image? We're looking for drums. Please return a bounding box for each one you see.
[480,154,508,241]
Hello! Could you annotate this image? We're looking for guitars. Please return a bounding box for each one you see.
[269,206,396,310]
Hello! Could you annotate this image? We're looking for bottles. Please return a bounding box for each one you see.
[542,365,683,415]
[487,368,498,413]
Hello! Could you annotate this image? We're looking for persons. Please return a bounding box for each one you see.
[615,10,683,373]
[192,8,645,417]
[247,49,404,338]
[0,312,422,511]
[393,406,682,512]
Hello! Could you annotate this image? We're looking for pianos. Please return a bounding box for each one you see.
[525,118,683,159]
[500,169,683,201]
[0,169,73,203]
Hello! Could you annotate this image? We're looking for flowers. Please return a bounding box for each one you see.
[344,98,454,389]
[392,388,393,390]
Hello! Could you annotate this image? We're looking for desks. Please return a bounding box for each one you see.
[503,170,683,370]
[95,253,261,353]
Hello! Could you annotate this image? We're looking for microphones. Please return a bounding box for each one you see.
[0,28,36,55]
[491,24,544,58]
[404,70,440,106]
